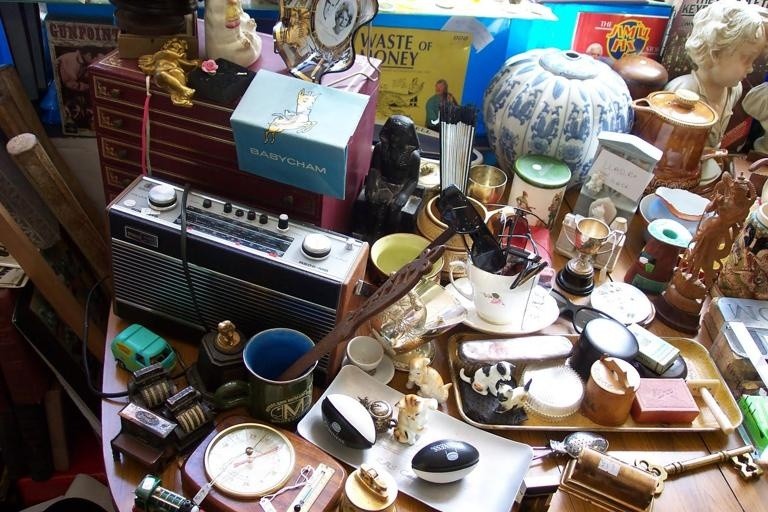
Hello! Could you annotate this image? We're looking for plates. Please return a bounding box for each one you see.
[341,355,395,386]
[442,278,559,337]
[296,365,533,512]
[590,280,651,327]
[344,468,398,512]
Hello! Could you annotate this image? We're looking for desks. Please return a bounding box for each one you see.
[100,187,768,512]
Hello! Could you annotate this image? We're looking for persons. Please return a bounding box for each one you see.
[84,53,105,130]
[681,179,758,291]
[139,38,197,108]
[56,47,93,133]
[425,79,456,130]
[365,115,421,208]
[333,2,354,33]
[666,0,766,179]
[586,43,603,60]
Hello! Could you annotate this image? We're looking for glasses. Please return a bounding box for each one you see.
[435,183,508,274]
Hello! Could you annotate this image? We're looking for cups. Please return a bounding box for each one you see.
[211,328,318,424]
[448,253,538,326]
[347,336,384,373]
[466,163,507,208]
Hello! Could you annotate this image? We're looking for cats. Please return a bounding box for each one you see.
[393,394,424,445]
[407,356,452,403]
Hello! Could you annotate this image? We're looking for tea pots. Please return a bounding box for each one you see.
[630,89,719,213]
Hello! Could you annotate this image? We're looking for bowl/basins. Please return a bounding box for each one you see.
[639,191,704,247]
[370,233,444,284]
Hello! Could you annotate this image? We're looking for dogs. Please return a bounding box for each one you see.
[460,361,515,397]
[494,378,532,411]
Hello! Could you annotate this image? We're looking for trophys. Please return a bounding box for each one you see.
[556,218,625,296]
